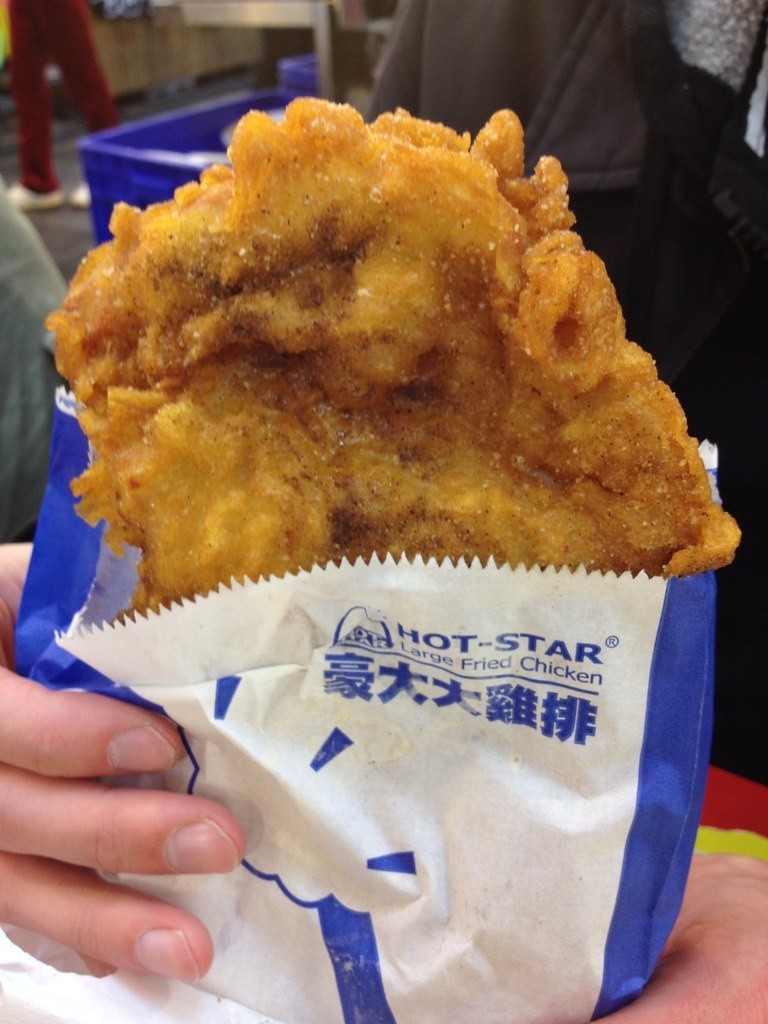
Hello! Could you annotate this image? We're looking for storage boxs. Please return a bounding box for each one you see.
[77,88,314,249]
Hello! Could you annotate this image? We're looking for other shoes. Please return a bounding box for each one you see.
[8,182,65,211]
[68,182,92,208]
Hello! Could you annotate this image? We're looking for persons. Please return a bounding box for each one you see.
[360,0,650,193]
[572,2,768,784]
[2,184,244,983]
[9,0,121,209]
